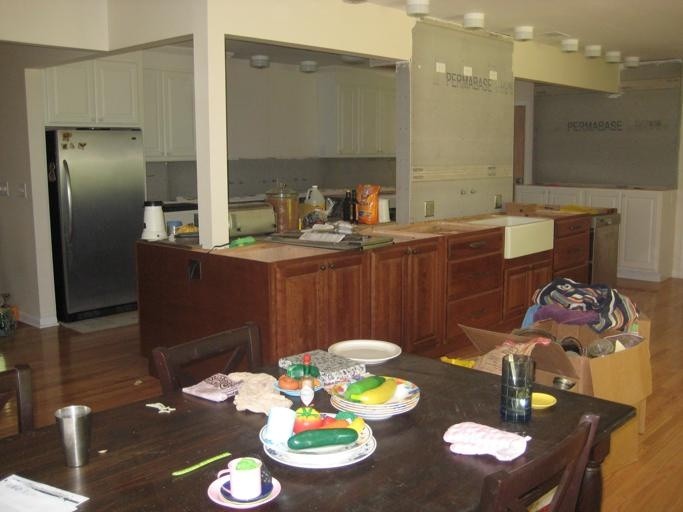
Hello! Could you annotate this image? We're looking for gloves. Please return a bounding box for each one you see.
[443,421,532,463]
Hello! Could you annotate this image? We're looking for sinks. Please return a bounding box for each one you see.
[467,215,553,228]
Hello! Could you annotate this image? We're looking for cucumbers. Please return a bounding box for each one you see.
[288,428,358,450]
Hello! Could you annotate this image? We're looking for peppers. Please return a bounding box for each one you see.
[344,374,385,398]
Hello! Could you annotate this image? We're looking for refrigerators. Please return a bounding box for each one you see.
[48,128,147,324]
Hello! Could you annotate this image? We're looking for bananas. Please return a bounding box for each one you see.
[350,379,397,405]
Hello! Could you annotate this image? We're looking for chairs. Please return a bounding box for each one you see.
[0,365,34,435]
[151,322,262,394]
[480,412,600,512]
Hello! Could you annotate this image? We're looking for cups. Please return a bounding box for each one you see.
[502,354,536,424]
[51,405,91,468]
[216,456,263,503]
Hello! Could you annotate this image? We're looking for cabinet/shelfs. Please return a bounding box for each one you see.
[505,251,554,330]
[272,234,369,381]
[555,212,589,285]
[140,71,193,162]
[357,82,399,154]
[335,81,357,155]
[44,51,140,128]
[370,223,445,353]
[445,219,504,341]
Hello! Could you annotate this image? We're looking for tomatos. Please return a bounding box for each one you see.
[294,407,322,433]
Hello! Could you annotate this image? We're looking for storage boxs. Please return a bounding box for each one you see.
[527,308,651,436]
[454,322,647,468]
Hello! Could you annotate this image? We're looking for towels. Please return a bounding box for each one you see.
[182,373,241,402]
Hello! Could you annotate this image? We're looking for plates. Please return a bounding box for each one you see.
[531,393,558,409]
[259,412,373,454]
[330,376,421,420]
[328,339,403,366]
[263,437,378,470]
[207,474,281,509]
[272,379,324,397]
